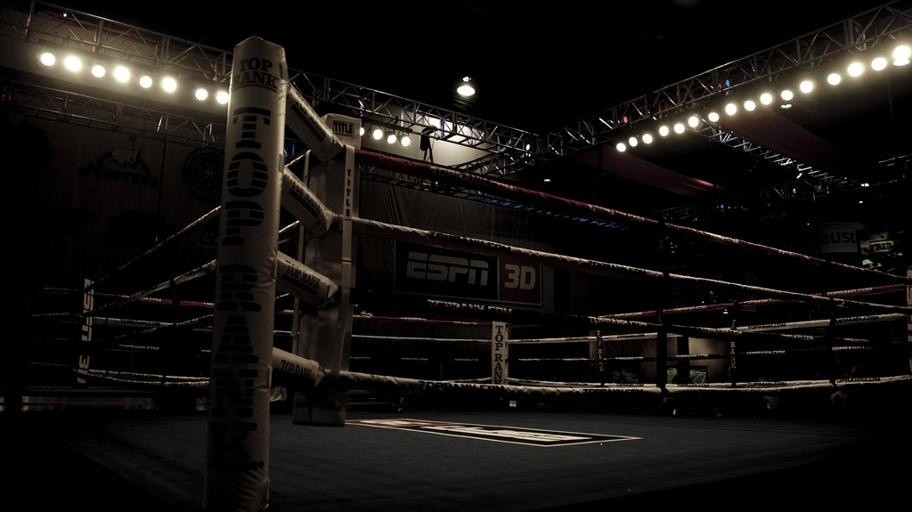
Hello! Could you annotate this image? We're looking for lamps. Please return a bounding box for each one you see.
[455,73,478,97]
[358,115,415,148]
